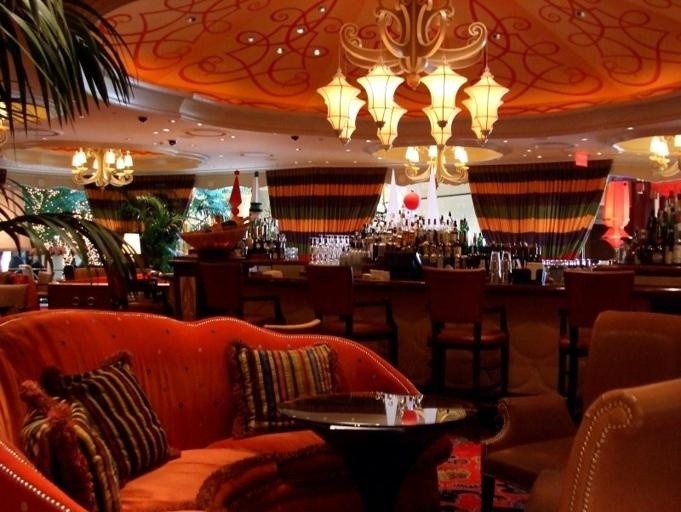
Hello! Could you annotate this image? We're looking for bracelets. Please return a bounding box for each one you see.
[180,224,249,251]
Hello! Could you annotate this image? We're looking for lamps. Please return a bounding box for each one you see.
[642,133,680,179]
[400,143,468,185]
[308,0,509,148]
[69,145,133,189]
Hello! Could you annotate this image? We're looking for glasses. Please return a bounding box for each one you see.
[642,133,680,179]
[69,145,133,189]
[308,0,509,148]
[400,143,468,185]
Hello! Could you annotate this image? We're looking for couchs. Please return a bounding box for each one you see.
[0,309,452,511]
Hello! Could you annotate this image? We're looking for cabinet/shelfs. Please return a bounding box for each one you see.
[616,206,681,265]
[243,217,298,261]
[308,210,598,286]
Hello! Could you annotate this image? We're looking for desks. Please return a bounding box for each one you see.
[277,392,468,511]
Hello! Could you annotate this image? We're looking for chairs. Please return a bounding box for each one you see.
[422,266,512,440]
[557,268,636,415]
[0,258,402,367]
[478,309,681,511]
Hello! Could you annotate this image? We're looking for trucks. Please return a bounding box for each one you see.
[403,192,420,211]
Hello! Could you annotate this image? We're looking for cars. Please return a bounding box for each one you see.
[381,393,437,426]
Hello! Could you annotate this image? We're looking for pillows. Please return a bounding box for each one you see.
[22,378,123,511]
[231,344,339,438]
[42,350,182,487]
